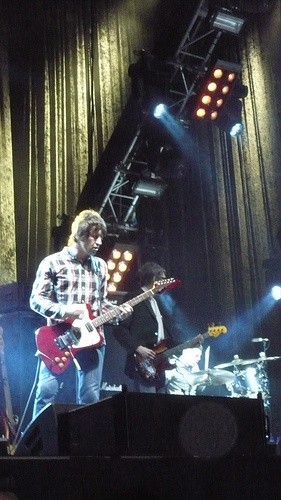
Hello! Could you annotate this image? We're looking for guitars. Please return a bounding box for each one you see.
[133,321,229,384]
[34,276,181,376]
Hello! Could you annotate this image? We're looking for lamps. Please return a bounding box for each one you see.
[150,6,244,138]
[106,243,138,292]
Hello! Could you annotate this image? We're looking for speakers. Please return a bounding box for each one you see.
[12,402,87,456]
[56,389,266,457]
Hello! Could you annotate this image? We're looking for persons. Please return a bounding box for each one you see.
[30,210,134,421]
[112,263,204,395]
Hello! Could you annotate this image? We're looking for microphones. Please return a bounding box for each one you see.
[252,337,269,342]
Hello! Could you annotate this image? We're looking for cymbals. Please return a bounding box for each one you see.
[214,359,257,369]
[255,356,281,362]
[189,368,235,385]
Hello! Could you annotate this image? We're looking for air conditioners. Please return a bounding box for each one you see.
[132,178,167,201]
